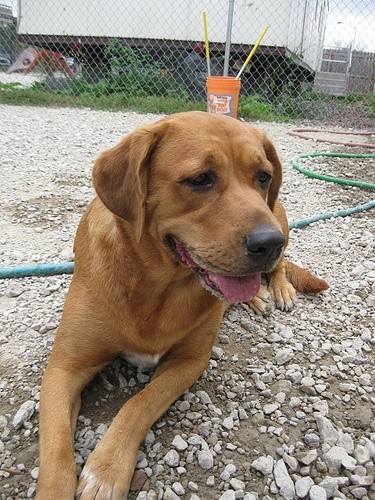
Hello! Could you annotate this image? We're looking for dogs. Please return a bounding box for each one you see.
[36,109,331,500]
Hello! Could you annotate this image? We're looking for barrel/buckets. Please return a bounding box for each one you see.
[206,76,241,120]
